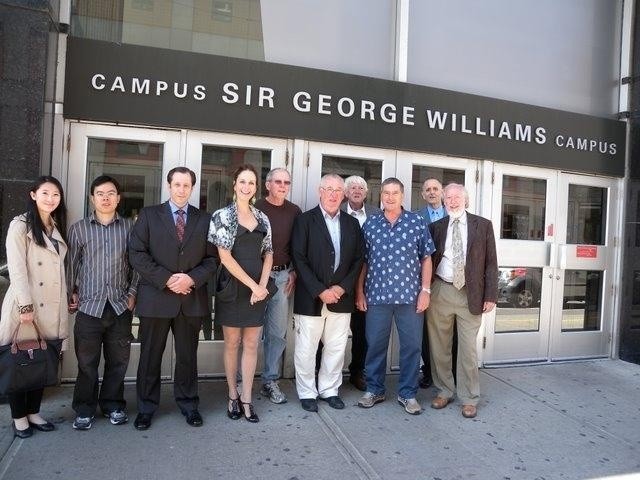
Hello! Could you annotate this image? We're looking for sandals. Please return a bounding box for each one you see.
[238,393,259,422]
[227,396,241,420]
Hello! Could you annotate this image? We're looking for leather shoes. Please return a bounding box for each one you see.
[12,420,33,438]
[29,416,54,432]
[181,411,203,426]
[133,412,155,430]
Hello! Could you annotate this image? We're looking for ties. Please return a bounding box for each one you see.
[175,209,186,241]
[452,219,466,290]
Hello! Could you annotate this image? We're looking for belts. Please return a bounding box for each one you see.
[270,262,291,272]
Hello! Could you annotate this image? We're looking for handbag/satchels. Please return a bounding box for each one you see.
[1,320,62,396]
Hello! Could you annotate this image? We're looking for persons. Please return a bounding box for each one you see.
[128,167,220,429]
[413,177,458,389]
[425,183,498,418]
[207,165,274,422]
[64,175,142,428]
[289,174,366,410]
[317,175,382,392]
[355,177,437,416]
[0,175,68,439]
[237,167,302,404]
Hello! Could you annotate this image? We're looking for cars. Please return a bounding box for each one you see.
[498,267,587,308]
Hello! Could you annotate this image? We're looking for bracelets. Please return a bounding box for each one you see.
[422,287,431,293]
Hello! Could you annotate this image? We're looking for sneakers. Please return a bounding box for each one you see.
[72,415,94,429]
[103,410,128,425]
[261,380,287,404]
[301,375,476,419]
